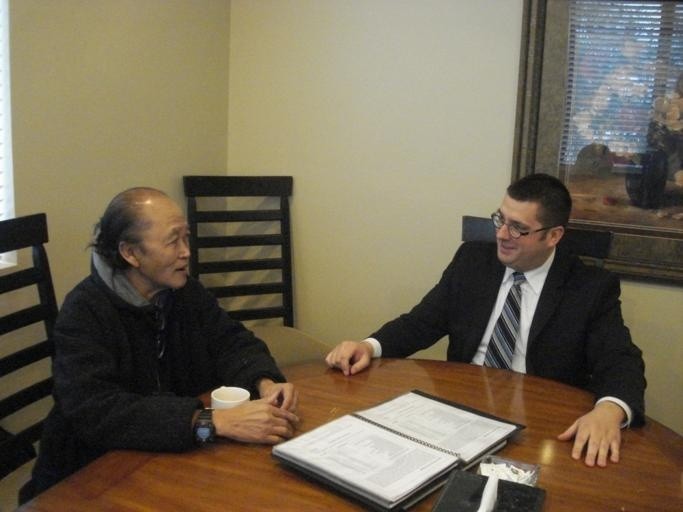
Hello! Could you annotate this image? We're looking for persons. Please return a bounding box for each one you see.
[18,185,301,506]
[325,174,646,469]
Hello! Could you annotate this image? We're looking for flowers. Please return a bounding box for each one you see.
[572,40,681,164]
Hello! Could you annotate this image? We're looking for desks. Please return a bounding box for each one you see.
[21,356,682,512]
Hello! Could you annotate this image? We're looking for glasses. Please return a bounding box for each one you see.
[491,212,562,239]
[153,311,166,360]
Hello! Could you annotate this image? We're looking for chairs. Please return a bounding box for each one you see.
[452,215,609,267]
[0,211,59,508]
[181,173,296,332]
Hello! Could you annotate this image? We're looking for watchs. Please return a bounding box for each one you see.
[193,407,217,445]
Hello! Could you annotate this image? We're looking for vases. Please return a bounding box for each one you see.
[625,141,670,209]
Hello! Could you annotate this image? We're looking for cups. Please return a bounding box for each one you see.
[210,385,250,409]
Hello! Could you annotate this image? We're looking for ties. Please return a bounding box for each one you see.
[483,271,526,369]
